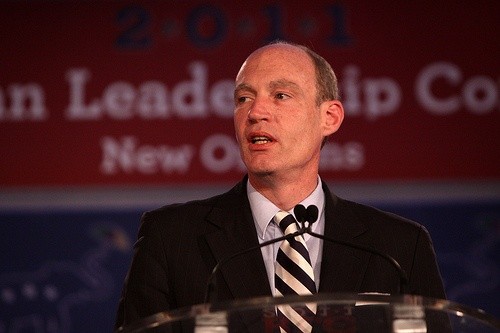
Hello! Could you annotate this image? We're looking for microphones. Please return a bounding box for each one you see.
[205,204,412,305]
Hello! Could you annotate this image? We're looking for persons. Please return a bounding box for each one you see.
[115,41,454,333]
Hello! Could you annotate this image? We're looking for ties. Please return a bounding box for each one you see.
[271,210,317,333]
